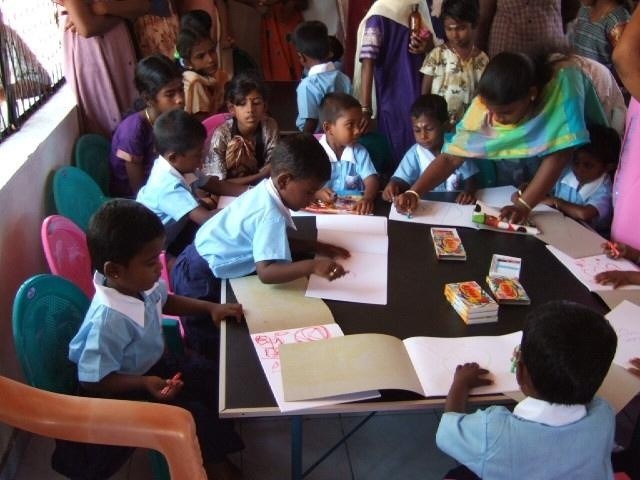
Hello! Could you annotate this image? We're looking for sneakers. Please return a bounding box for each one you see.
[205,457,244,480]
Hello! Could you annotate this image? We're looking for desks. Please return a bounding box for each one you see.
[218,191,640,480]
[265,80,302,136]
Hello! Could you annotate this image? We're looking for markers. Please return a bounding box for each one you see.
[160,372,184,398]
[607,240,621,256]
[406,199,410,218]
[484,218,543,236]
[510,345,519,372]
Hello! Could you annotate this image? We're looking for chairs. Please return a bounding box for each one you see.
[356,131,395,190]
[443,132,497,190]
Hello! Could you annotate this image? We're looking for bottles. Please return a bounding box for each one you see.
[409,3,422,43]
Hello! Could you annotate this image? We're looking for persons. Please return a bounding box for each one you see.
[53,1,639,478]
